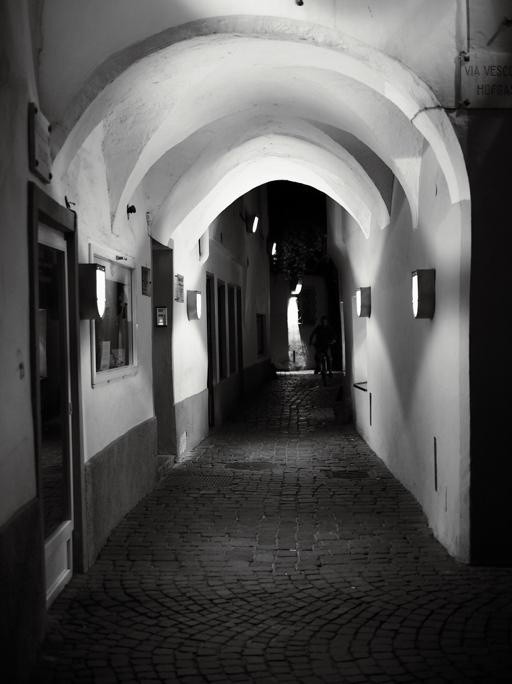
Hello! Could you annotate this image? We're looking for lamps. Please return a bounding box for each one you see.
[188,290,201,320]
[355,287,370,317]
[78,264,106,320]
[412,269,435,320]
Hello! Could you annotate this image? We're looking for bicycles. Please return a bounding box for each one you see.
[307,342,336,380]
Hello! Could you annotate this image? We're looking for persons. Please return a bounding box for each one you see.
[309,317,336,378]
